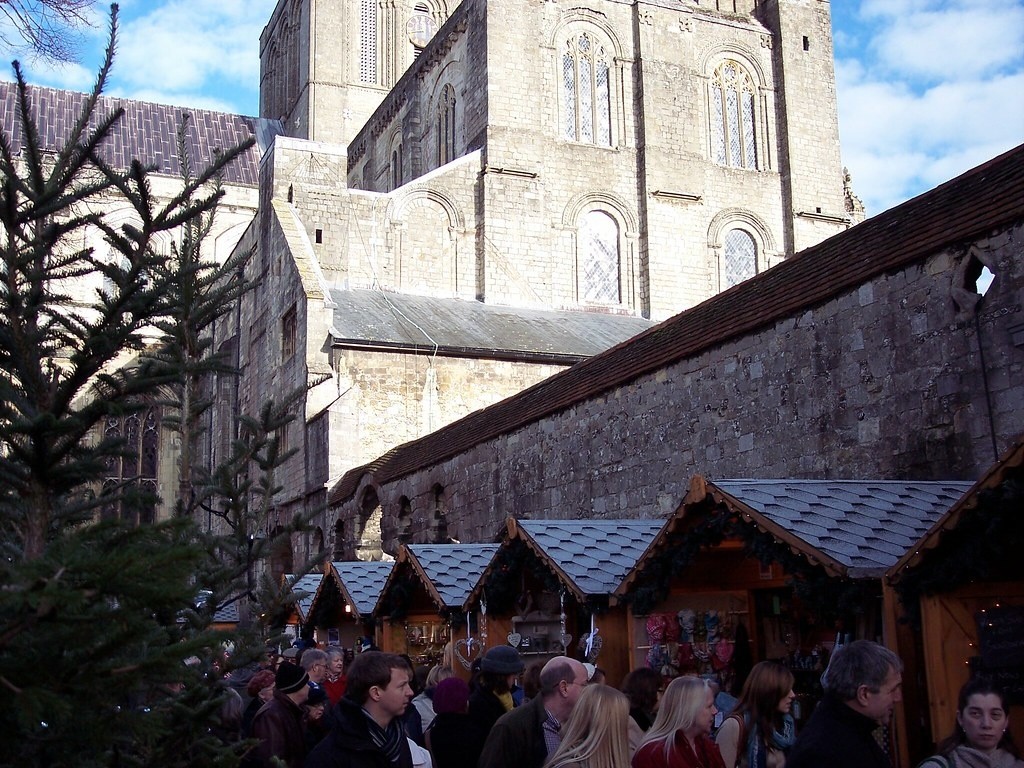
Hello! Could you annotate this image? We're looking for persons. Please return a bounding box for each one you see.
[160,640,1023,768]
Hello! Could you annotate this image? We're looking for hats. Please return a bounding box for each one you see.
[480,645,526,674]
[247,669,276,697]
[432,677,469,716]
[275,660,310,694]
[283,648,300,658]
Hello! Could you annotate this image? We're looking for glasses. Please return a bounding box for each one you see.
[554,680,588,690]
[311,663,328,669]
